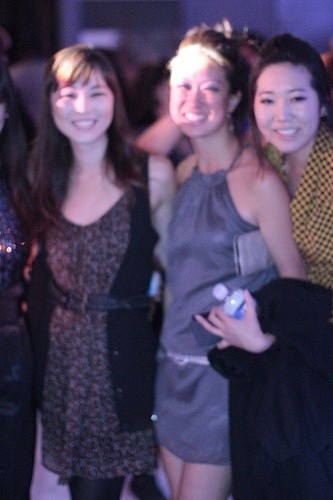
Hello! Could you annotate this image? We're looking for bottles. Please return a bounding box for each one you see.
[213,283,259,321]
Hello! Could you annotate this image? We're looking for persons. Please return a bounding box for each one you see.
[0,49,37,500]
[24,44,173,500]
[0,28,333,179]
[154,29,306,499]
[176,32,333,289]
